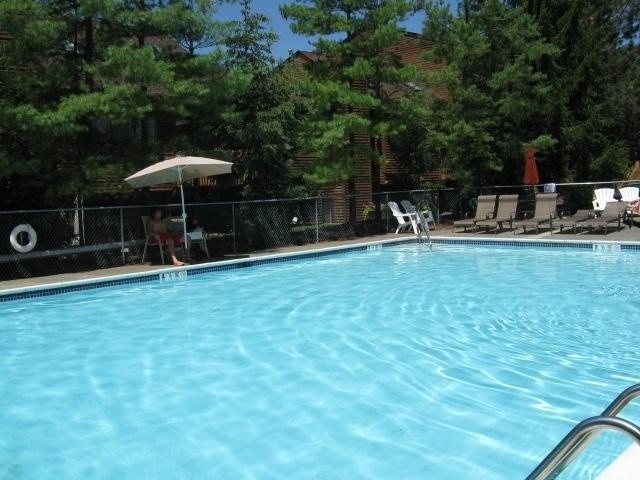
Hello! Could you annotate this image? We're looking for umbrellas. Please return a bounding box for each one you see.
[523,145,541,192]
[122,150,236,252]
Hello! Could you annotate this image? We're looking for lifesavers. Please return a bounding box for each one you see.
[9,223,37,254]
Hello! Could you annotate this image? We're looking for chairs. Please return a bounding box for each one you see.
[453,186,640,236]
[140,215,211,266]
[387,200,436,236]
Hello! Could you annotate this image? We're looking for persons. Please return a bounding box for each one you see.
[146,207,193,266]
